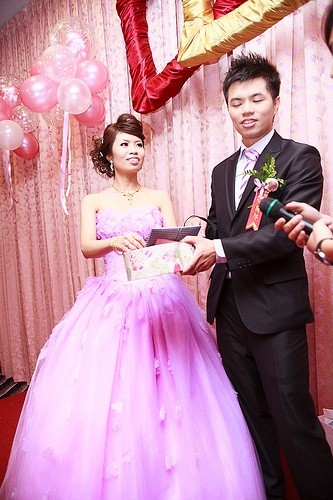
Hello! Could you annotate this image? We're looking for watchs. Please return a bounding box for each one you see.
[314,238,333,265]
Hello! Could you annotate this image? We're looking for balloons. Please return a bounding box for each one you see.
[41,44,77,82]
[76,59,107,93]
[0,120,23,150]
[57,78,92,114]
[73,93,105,127]
[13,133,39,159]
[19,75,59,113]
[0,75,38,133]
[30,31,90,75]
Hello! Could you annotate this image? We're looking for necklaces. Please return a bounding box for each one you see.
[112,183,143,203]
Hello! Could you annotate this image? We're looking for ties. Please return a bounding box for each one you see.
[238,149,260,206]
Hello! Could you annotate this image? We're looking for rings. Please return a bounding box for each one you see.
[125,243,129,246]
[131,239,135,243]
[196,270,199,274]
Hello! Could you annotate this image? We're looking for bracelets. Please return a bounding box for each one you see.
[112,236,124,256]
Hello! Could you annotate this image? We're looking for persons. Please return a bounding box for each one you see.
[0,113,266,500]
[274,0,333,266]
[180,51,333,500]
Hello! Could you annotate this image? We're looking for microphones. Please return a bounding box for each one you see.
[259,197,314,236]
